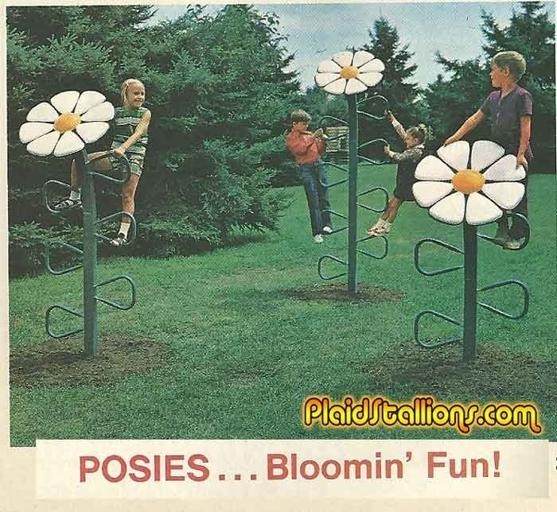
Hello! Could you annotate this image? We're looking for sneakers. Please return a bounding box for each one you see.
[495,223,510,245]
[314,227,333,245]
[365,225,391,236]
[109,232,129,248]
[504,226,525,251]
[53,198,83,211]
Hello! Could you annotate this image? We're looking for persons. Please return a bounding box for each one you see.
[52,78,152,248]
[366,108,425,237]
[442,50,533,250]
[286,109,333,243]
